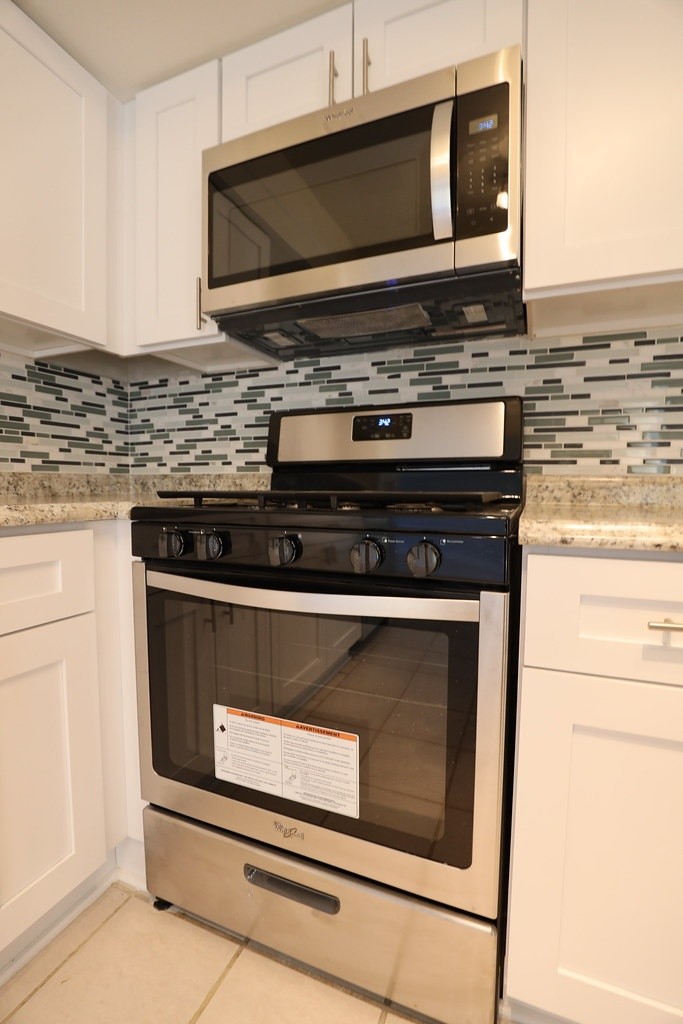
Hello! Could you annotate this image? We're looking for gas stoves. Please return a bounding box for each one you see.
[132,397,527,588]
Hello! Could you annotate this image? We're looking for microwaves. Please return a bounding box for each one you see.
[198,45,525,361]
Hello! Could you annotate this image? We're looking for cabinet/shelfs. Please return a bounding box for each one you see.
[0,516,126,989]
[1,0,124,360]
[269,524,362,720]
[124,57,279,378]
[146,526,269,793]
[520,0,683,340]
[220,0,525,144]
[494,544,682,1024]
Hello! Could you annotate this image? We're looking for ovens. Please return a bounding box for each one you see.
[126,574,511,923]
